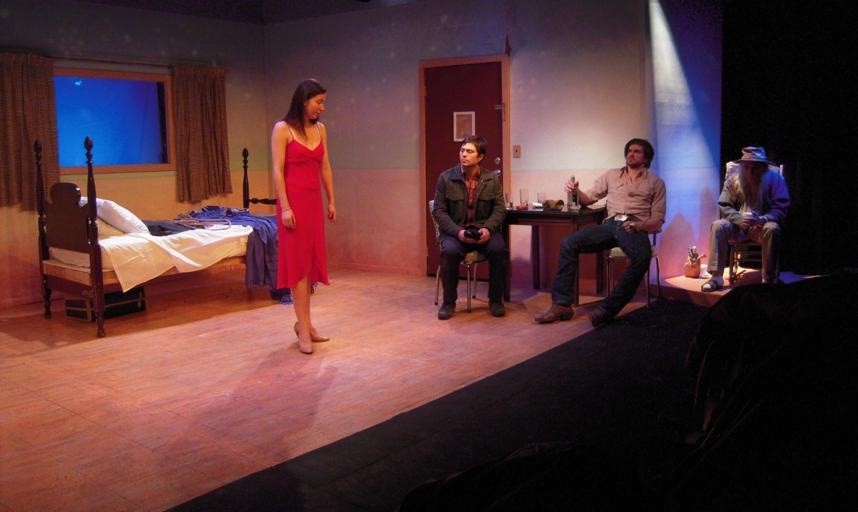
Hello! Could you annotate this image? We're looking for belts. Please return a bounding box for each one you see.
[610,213,641,222]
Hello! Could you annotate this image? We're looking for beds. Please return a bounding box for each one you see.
[30,137,318,340]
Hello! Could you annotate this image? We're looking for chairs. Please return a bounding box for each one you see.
[609,224,662,313]
[427,197,488,315]
[719,159,786,285]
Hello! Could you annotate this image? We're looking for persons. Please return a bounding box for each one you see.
[433,134,509,321]
[396,282,858,512]
[534,137,667,324]
[701,146,792,293]
[270,77,337,356]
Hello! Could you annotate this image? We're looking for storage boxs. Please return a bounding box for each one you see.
[61,284,148,324]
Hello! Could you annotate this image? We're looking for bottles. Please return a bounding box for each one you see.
[567,177,579,211]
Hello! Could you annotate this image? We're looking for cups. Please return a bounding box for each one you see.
[537,192,547,203]
[743,213,753,218]
[504,191,514,210]
[520,189,529,206]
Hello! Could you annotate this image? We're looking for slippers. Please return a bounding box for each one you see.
[702,277,726,292]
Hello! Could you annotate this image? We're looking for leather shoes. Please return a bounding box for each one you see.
[588,304,608,328]
[532,304,573,323]
[488,297,505,317]
[437,300,457,318]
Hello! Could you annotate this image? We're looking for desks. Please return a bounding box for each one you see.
[497,203,606,310]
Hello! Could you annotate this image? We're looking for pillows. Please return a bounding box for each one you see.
[75,191,152,238]
[86,215,127,243]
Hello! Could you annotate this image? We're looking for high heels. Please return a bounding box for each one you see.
[293,322,332,354]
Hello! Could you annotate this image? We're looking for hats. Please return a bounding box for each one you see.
[736,145,776,168]
